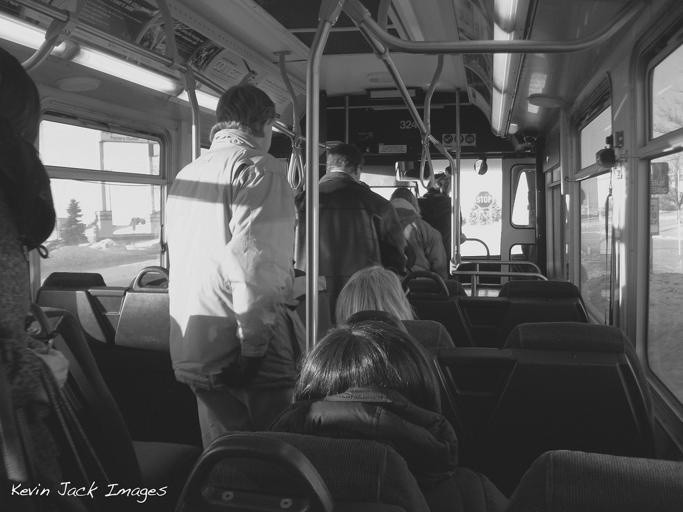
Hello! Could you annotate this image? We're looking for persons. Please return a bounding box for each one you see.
[294,141,405,338]
[417,173,464,257]
[334,266,417,324]
[266,323,516,512]
[390,186,447,280]
[1,46,74,494]
[164,84,298,450]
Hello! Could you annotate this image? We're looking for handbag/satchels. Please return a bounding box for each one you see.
[22,302,140,507]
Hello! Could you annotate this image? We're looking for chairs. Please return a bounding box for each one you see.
[26,254,683,512]
